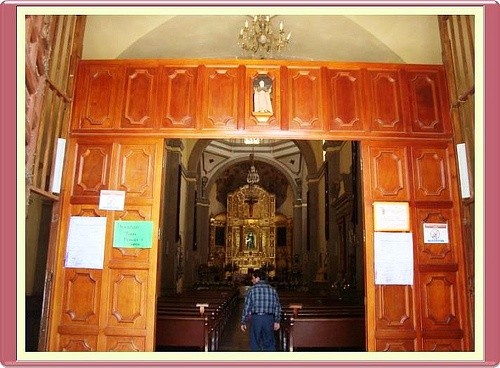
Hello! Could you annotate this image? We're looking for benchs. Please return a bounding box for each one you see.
[155,284,367,352]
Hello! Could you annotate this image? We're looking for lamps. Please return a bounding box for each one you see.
[237,15,292,59]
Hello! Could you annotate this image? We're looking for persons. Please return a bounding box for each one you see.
[240,269,283,352]
